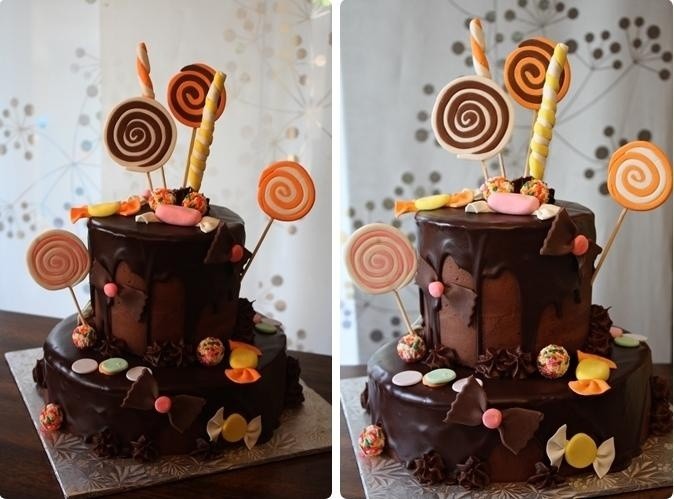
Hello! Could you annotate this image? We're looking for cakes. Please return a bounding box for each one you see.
[343,17,673,489]
[26,40,316,464]
